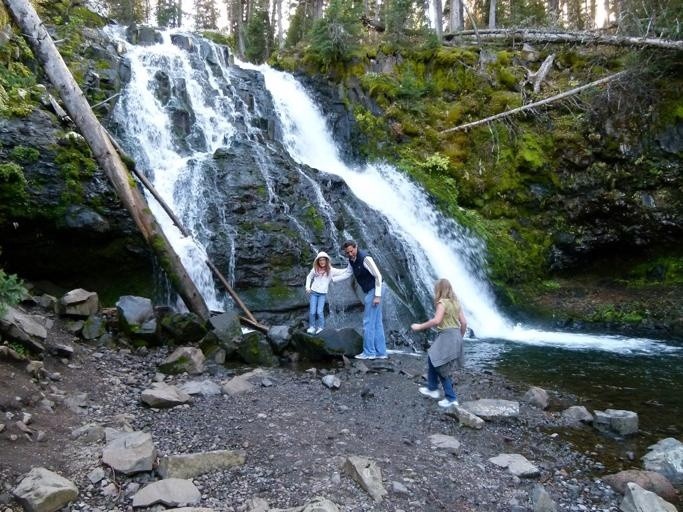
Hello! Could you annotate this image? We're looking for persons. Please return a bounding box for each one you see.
[410,277,466,409]
[329,239,389,361]
[304,251,348,335]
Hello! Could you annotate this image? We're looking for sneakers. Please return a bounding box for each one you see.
[418,387,439,398]
[438,398,459,407]
[354,353,389,359]
[306,327,322,334]
